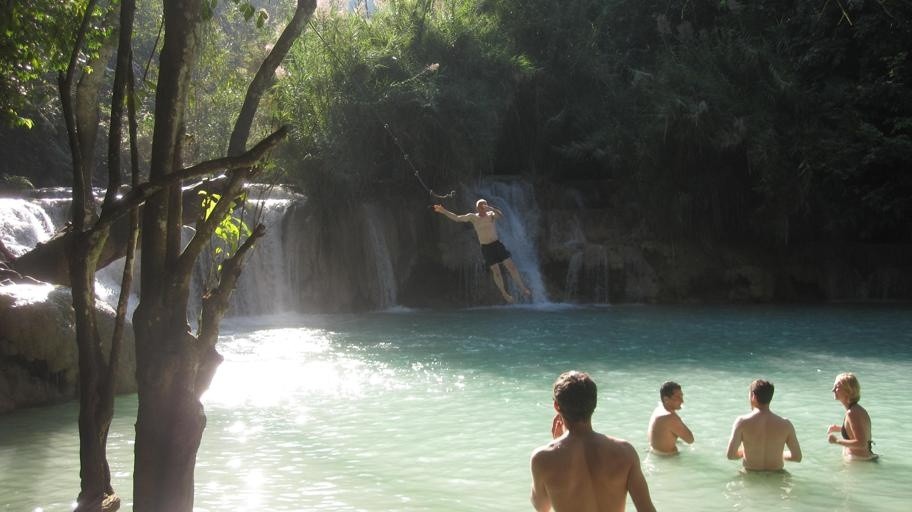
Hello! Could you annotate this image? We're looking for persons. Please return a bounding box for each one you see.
[528,368,660,512]
[726,378,803,473]
[648,380,694,457]
[433,196,532,304]
[827,371,880,463]
